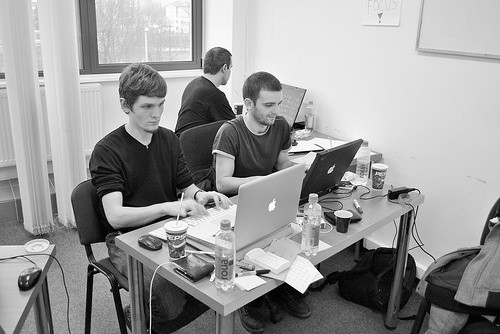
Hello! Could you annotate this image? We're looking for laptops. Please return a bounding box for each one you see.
[276,84,306,131]
[180,139,364,252]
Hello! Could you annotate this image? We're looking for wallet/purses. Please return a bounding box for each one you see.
[173,253,214,283]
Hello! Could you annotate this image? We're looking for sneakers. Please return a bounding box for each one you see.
[238,299,265,334]
[272,285,312,318]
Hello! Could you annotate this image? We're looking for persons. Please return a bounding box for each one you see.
[174,46,236,139]
[89,64,233,332]
[212,72,311,334]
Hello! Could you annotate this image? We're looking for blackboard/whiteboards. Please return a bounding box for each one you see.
[415,0,500,60]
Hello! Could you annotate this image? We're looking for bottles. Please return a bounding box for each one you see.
[214,220,236,291]
[304,100,315,132]
[299,193,323,256]
[355,141,370,185]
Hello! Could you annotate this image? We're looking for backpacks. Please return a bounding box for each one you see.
[327,247,420,315]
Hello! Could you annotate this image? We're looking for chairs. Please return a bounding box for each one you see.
[410,197,500,334]
[71,179,129,334]
[179,121,228,195]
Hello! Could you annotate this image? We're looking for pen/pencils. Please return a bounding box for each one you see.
[353,199,363,214]
[235,270,270,277]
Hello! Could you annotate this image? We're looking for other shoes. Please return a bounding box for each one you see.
[123,304,159,334]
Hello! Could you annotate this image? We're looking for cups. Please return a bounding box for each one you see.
[334,210,353,233]
[163,220,188,260]
[371,163,388,191]
[234,104,243,115]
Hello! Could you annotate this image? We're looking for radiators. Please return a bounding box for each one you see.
[0,82,103,166]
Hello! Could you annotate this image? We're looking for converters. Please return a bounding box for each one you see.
[388,186,409,200]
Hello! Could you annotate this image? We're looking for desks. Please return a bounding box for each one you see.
[290,128,382,173]
[114,179,424,334]
[0,243,57,334]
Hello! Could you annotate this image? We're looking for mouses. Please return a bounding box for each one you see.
[17,267,42,291]
[137,234,163,251]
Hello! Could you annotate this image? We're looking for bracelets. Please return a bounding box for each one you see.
[194,189,204,200]
